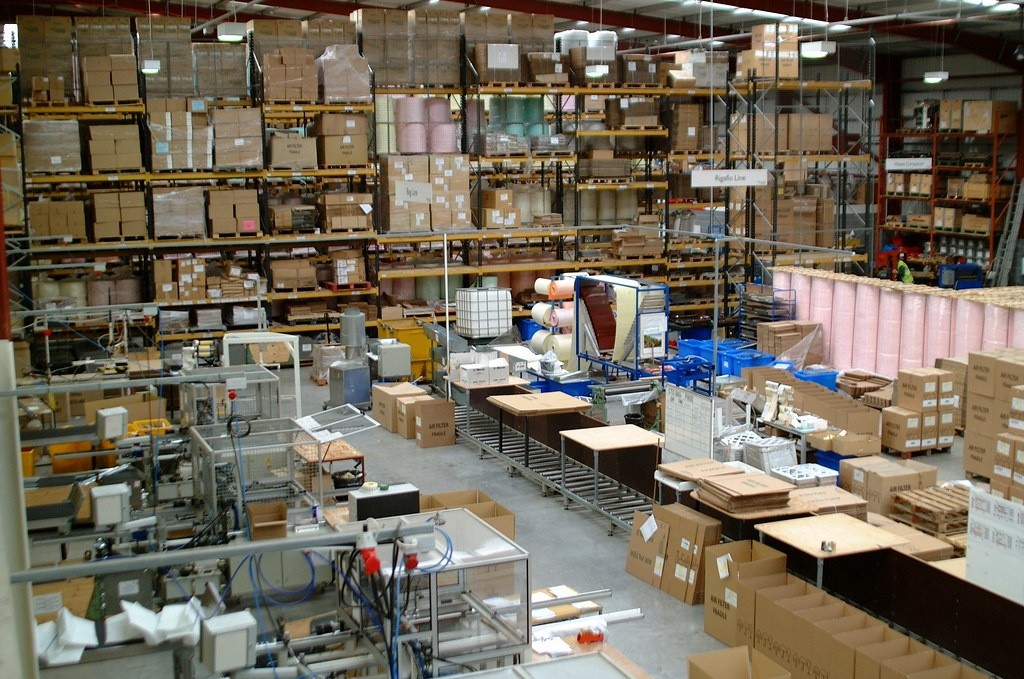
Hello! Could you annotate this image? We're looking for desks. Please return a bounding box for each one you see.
[480,584,604,629]
[654,460,765,505]
[442,375,531,435]
[558,424,665,502]
[753,512,911,590]
[487,391,594,468]
[278,429,365,492]
[689,491,820,544]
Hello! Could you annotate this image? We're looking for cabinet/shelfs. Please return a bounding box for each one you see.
[1,99,379,367]
[875,114,1024,287]
[1,107,33,349]
[737,283,797,343]
[0,0,1024,679]
[371,80,872,324]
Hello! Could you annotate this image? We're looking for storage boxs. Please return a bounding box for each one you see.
[0,9,1024,679]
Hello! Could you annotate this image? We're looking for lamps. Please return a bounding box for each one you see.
[584,0,609,77]
[140,0,161,74]
[674,0,706,65]
[800,0,837,59]
[924,0,950,86]
[217,0,246,42]
